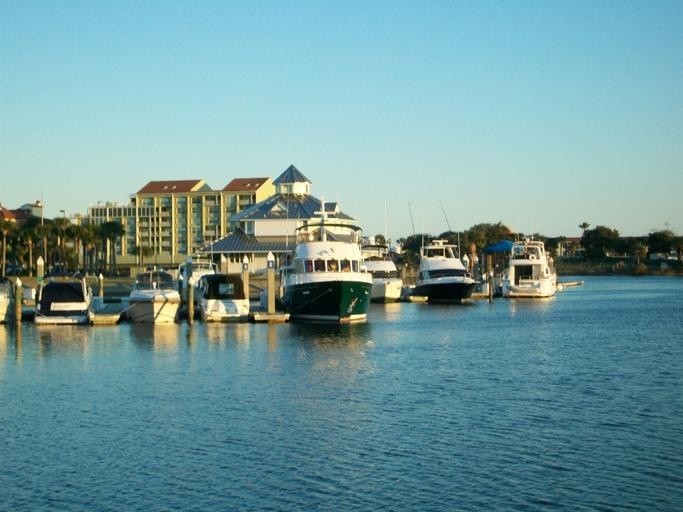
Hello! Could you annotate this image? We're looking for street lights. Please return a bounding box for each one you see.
[37,200,45,277]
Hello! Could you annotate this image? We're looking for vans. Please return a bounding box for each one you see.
[667,250,680,261]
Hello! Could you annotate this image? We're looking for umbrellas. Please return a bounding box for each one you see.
[483,240,523,252]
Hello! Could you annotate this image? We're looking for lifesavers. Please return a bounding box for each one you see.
[529,254,536,261]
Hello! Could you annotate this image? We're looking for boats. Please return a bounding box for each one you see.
[407,238,479,306]
[1,277,16,324]
[362,243,402,302]
[35,277,91,325]
[280,200,372,323]
[488,239,558,296]
[199,275,250,322]
[128,288,180,323]
